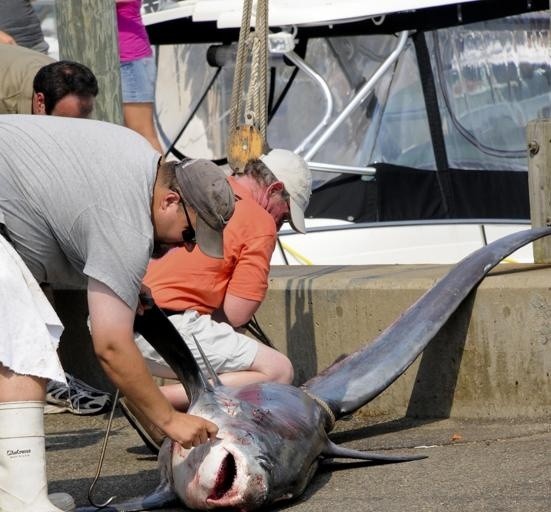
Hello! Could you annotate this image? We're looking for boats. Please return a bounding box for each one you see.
[141,1,551,267]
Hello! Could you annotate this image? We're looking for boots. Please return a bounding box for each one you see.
[1,401,75,511]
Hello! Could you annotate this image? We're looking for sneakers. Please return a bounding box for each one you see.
[44,373,109,416]
[119,397,161,451]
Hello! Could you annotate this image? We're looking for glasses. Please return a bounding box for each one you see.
[170,187,195,243]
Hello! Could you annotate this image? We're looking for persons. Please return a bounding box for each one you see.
[0,0,50,56]
[0,41,100,118]
[0,113,237,512]
[115,0,165,155]
[87,146,314,454]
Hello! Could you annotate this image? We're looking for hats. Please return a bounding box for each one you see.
[175,159,235,258]
[258,148,313,234]
